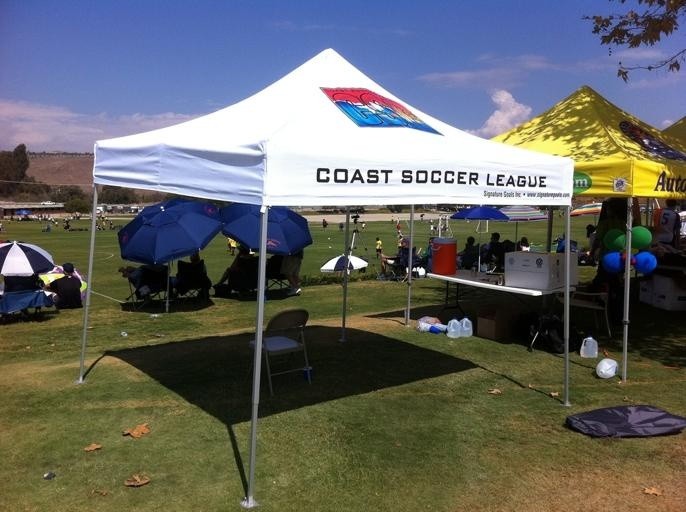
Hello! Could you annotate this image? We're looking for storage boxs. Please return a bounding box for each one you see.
[649,271,685,312]
[503,250,579,294]
[637,277,653,304]
[475,301,524,343]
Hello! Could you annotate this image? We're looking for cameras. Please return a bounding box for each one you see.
[351,215,360,218]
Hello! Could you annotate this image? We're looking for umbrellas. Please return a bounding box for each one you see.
[0,239,54,277]
[497,205,548,242]
[450,206,509,242]
[217,202,313,256]
[117,198,223,315]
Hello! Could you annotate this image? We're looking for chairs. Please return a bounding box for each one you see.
[242,303,316,398]
[224,256,267,298]
[550,271,613,339]
[266,252,295,296]
[124,264,169,313]
[381,245,418,283]
[168,259,206,306]
[2,276,42,324]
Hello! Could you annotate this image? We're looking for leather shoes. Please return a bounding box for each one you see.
[287,287,302,296]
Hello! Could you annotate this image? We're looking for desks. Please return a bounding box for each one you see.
[424,267,564,353]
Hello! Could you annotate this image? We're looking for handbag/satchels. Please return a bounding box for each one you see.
[279,256,289,275]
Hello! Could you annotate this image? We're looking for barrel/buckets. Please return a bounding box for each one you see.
[460,318,473,337]
[431,238,457,274]
[580,337,598,358]
[596,359,619,379]
[447,319,461,338]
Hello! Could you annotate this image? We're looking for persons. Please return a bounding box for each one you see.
[189,252,206,277]
[0,221,6,232]
[550,197,686,294]
[457,232,529,270]
[95,214,113,231]
[212,241,250,286]
[129,264,167,299]
[37,213,83,232]
[44,263,84,310]
[319,212,449,280]
[226,237,237,255]
[279,249,303,296]
[1,208,34,222]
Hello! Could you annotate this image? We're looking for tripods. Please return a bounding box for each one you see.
[340,223,385,286]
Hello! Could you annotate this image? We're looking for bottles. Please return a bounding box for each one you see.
[151,314,162,318]
[120,331,127,337]
[555,259,562,285]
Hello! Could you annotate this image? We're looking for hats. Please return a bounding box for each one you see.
[62,263,74,273]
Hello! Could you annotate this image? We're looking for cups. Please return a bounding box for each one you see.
[498,275,503,285]
[471,263,477,277]
